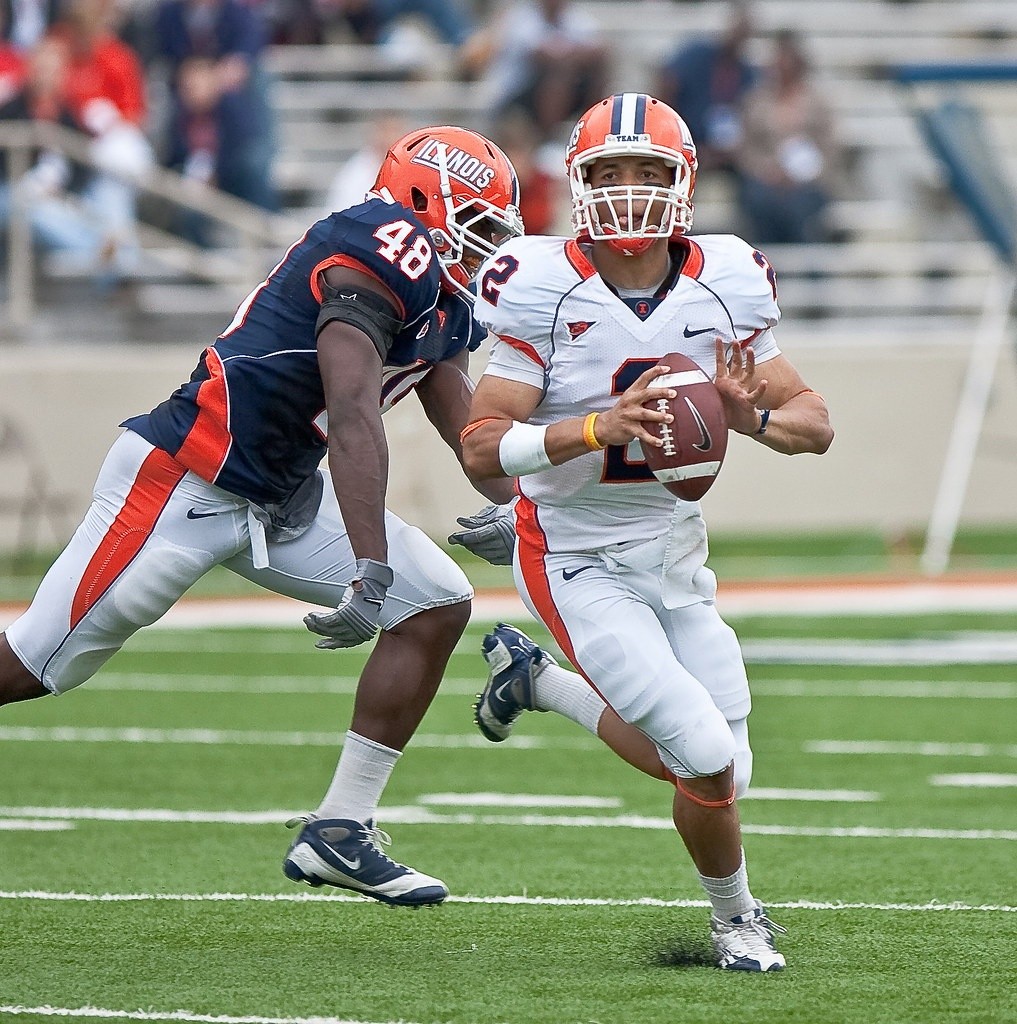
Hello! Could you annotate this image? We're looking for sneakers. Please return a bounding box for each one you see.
[471,622,559,743]
[281,813,450,910]
[709,897,788,971]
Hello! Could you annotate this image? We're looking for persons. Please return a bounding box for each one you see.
[0,0,854,341]
[460,92,835,973]
[0,125,525,910]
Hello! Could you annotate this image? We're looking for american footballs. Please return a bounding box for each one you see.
[638,352,730,503]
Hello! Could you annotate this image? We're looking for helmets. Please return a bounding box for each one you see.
[364,124,525,296]
[565,92,698,257]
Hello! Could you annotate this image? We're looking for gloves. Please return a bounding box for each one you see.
[303,557,394,649]
[448,492,523,566]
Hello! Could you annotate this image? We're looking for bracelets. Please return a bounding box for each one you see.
[733,408,771,436]
[582,411,607,451]
